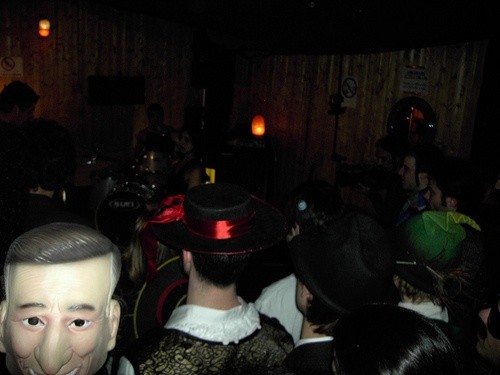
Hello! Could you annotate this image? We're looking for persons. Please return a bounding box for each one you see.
[0,79,500,375]
[139,184,296,375]
[0,220,135,374]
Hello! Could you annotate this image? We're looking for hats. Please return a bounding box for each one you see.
[145,179,286,254]
[382,211,480,294]
[287,208,395,311]
